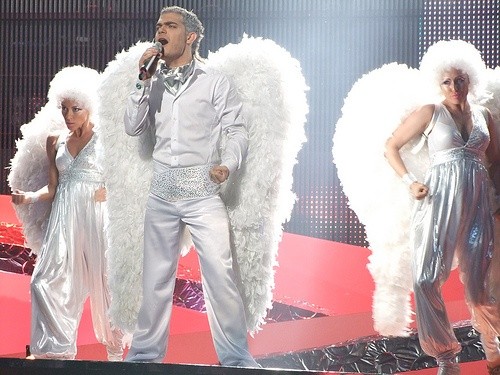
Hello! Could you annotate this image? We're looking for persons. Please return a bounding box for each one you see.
[95,6,311,369]
[330,39,500,375]
[4,65,128,362]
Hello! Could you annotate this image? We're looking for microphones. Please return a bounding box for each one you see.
[141,42,162,73]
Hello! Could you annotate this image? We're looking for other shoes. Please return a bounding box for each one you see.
[435,361,460,375]
[486,361,500,375]
